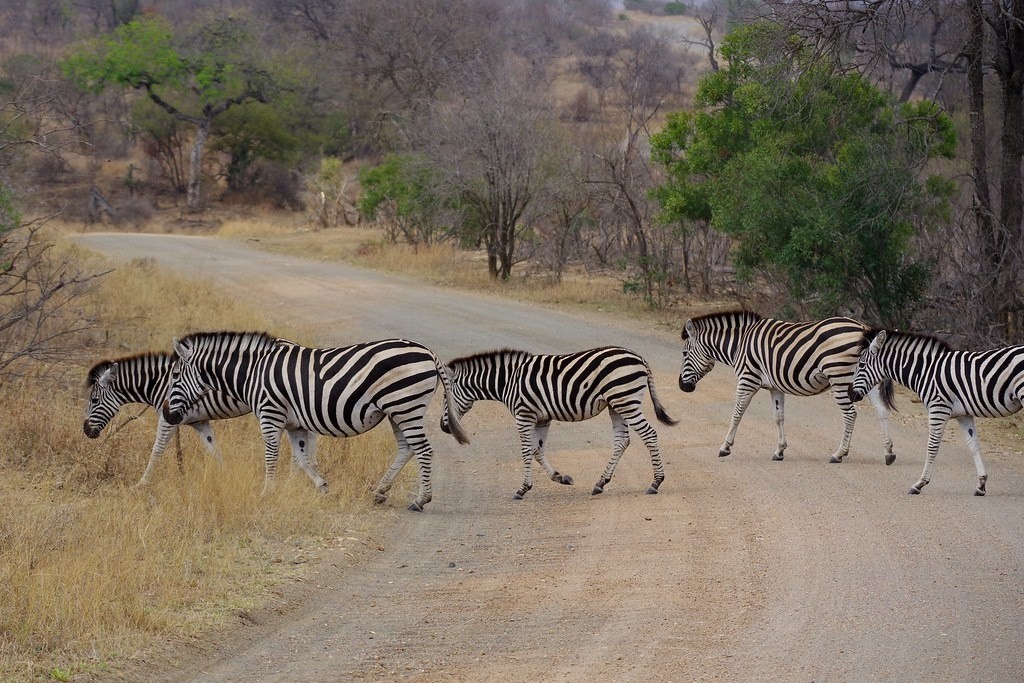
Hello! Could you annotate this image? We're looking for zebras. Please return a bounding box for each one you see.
[83,332,469,512]
[679,310,1023,499]
[439,345,675,502]
